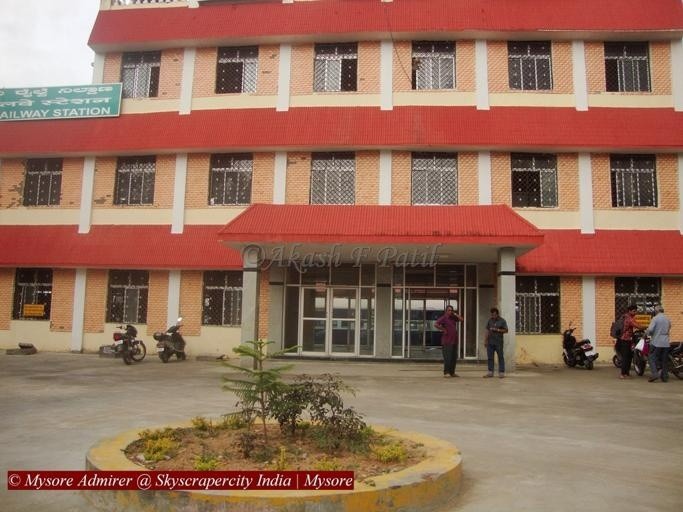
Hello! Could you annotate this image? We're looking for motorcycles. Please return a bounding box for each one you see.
[154,317,186,363]
[612,329,683,380]
[561,321,599,369]
[111,325,146,365]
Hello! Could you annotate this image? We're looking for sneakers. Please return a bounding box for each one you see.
[442,371,505,379]
[619,374,669,383]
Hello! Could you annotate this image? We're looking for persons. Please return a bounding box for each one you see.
[610,303,672,383]
[483,307,509,378]
[434,305,463,378]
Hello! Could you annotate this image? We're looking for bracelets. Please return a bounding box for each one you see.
[454,313,456,316]
[498,328,500,332]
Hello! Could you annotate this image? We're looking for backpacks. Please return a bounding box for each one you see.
[609,315,629,339]
